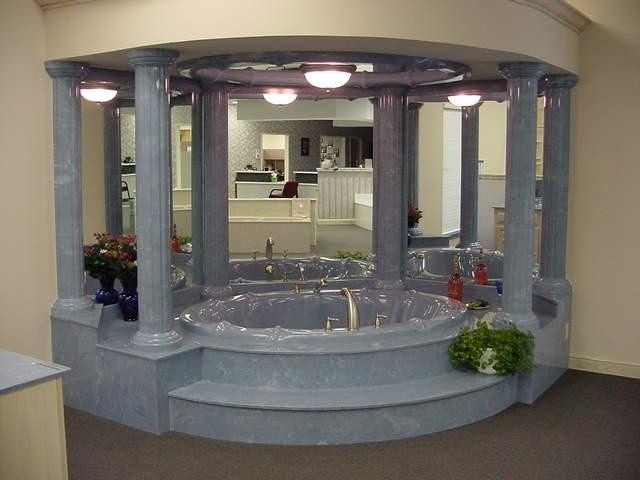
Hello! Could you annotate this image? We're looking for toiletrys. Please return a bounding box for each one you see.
[472,246,488,285]
[448,251,465,302]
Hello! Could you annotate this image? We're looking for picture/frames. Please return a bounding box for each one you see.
[301,137,310,156]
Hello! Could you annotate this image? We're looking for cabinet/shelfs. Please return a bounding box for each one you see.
[494,208,542,265]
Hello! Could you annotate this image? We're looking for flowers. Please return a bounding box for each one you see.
[81,230,138,280]
[407,200,423,229]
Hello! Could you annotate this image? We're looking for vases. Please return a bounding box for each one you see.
[407,233,411,248]
[95,276,139,321]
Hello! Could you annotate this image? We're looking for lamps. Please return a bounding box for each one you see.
[446,92,481,107]
[262,88,299,106]
[79,81,120,105]
[298,63,357,88]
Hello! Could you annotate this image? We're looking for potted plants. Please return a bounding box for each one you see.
[448,321,538,375]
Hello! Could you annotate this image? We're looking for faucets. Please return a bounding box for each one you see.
[312,279,328,293]
[295,263,307,282]
[340,287,360,332]
[408,251,425,261]
[265,237,274,260]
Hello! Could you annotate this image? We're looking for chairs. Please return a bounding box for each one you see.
[121,181,135,202]
[269,181,298,198]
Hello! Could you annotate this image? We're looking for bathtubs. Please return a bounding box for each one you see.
[408,245,505,285]
[227,257,377,287]
[181,290,469,387]
